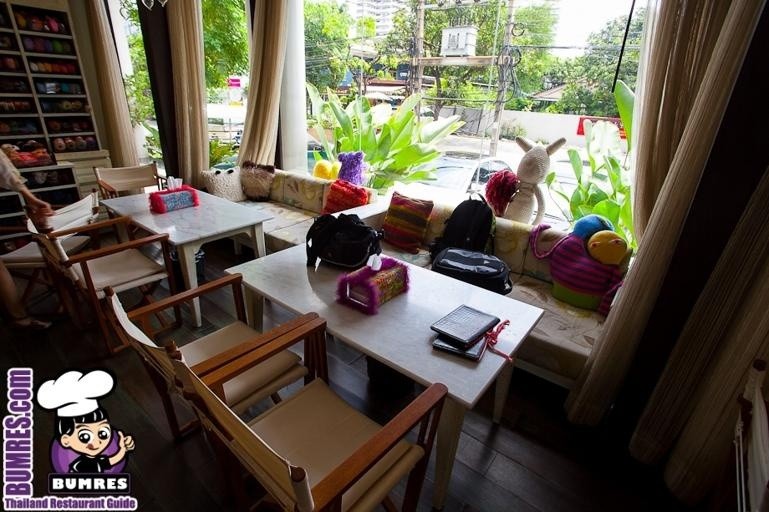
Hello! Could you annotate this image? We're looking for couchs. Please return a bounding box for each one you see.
[330,203,624,390]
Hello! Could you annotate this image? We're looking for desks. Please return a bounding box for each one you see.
[223,239,546,510]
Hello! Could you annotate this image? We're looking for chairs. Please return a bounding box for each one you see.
[22,160,449,512]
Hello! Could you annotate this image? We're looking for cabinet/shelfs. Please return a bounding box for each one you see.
[0,0,116,254]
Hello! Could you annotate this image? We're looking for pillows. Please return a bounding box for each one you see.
[379,191,436,253]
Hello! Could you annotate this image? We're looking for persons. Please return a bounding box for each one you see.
[0,146,57,330]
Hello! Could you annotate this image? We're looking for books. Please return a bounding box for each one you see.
[429,303,505,364]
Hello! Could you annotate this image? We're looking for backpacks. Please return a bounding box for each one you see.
[429,192,497,264]
[432,246,514,296]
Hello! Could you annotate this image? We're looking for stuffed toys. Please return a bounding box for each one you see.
[505,133,569,228]
[528,213,628,314]
[337,150,365,186]
[309,159,340,181]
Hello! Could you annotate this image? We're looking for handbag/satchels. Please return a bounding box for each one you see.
[306,211,386,272]
